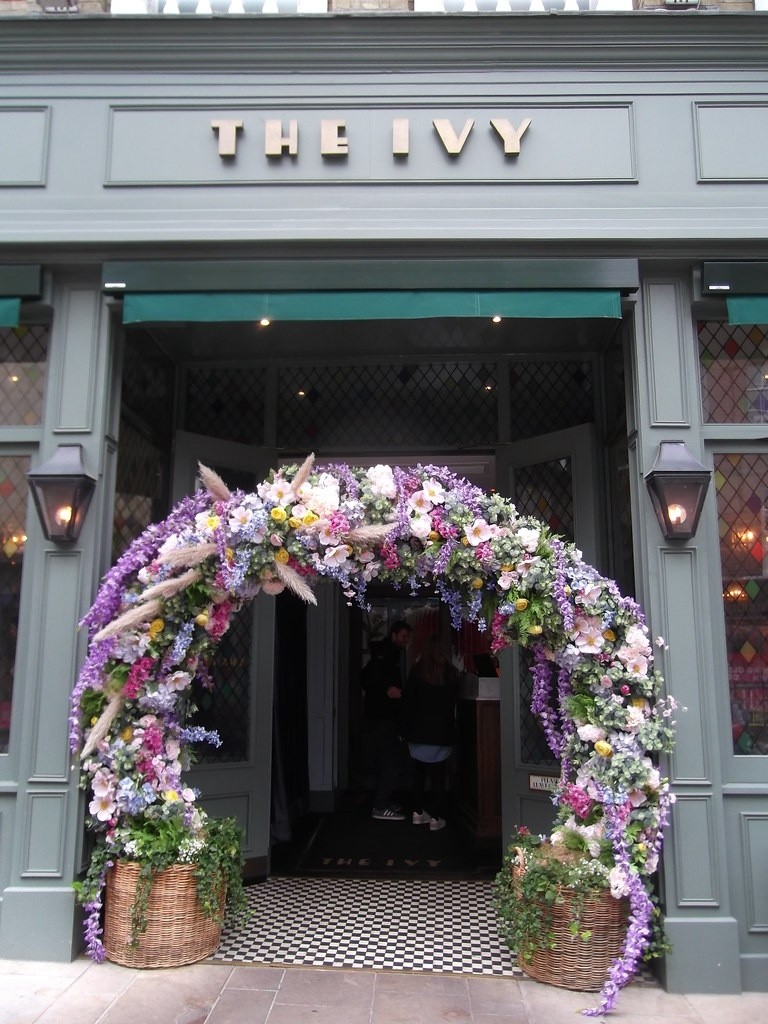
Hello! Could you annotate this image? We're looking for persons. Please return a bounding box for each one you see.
[362,624,464,830]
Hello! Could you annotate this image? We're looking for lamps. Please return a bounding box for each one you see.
[24,441,97,543]
[643,440,714,541]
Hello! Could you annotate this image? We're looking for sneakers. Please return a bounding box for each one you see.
[412,808,431,824]
[430,816,446,831]
[373,808,406,820]
[389,804,402,812]
